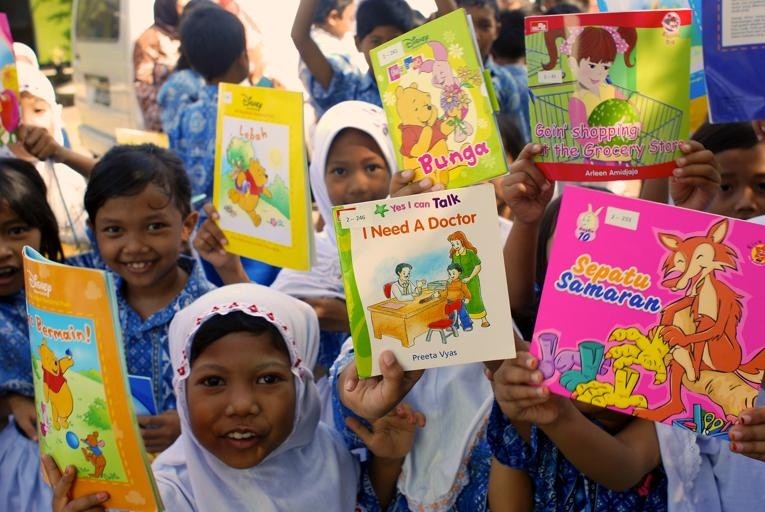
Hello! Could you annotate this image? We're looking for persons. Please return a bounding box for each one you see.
[0,0,765,512]
[42,282,426,512]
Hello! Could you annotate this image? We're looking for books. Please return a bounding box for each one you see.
[527,184,765,442]
[596,1,707,99]
[370,9,506,195]
[22,243,164,512]
[211,77,316,276]
[523,11,691,181]
[701,0,765,124]
[331,182,518,379]
